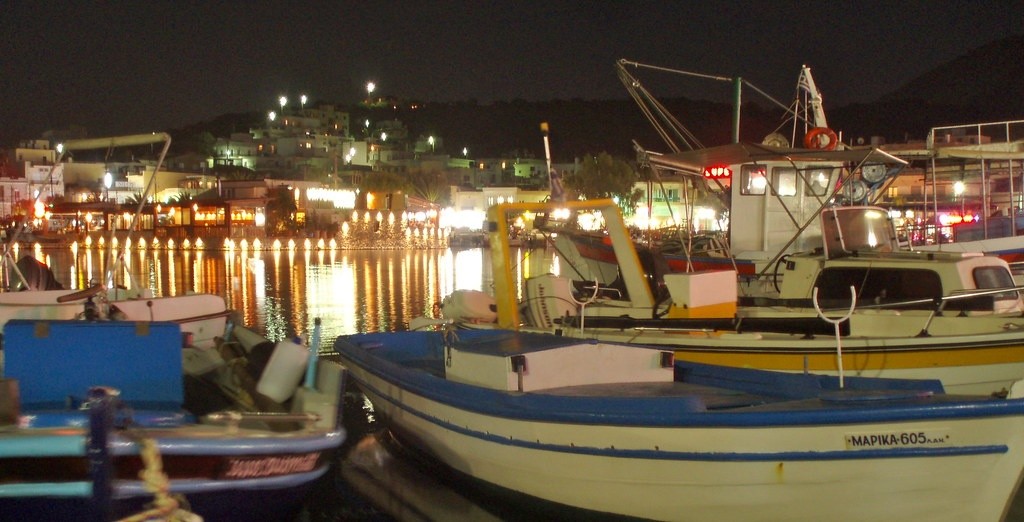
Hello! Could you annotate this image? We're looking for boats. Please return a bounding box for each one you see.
[408,59,1024,398]
[333,329,1024,522]
[0,132,347,498]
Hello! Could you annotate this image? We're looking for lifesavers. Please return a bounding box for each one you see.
[805,126,838,151]
